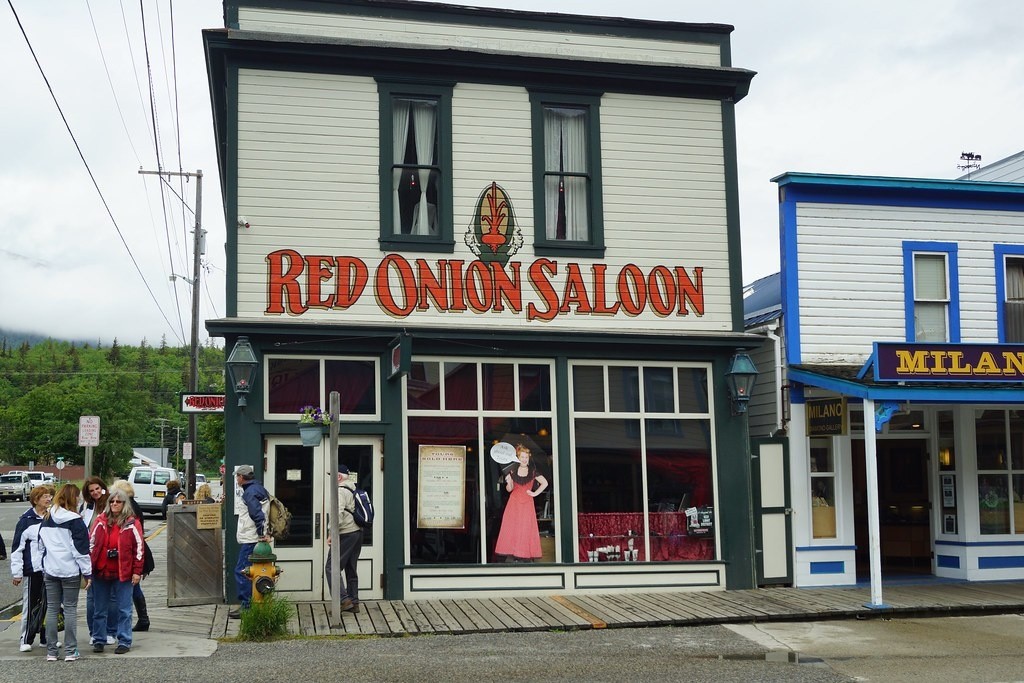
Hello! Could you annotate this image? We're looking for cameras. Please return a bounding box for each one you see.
[107,548,118,558]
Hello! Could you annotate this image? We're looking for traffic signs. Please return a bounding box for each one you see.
[179,392,226,414]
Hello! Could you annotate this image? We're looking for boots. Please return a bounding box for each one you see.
[132,597,150,631]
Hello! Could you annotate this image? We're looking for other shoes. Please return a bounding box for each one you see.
[347,604,359,613]
[89,637,95,645]
[329,600,354,614]
[94,643,104,653]
[20,644,32,652]
[47,653,59,660]
[39,642,62,648]
[114,645,129,654]
[228,607,241,618]
[65,652,80,661]
[106,636,115,644]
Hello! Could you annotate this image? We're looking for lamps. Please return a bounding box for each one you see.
[724,347,759,416]
[227,335,260,413]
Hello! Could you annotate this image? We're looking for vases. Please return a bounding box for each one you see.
[300,427,323,447]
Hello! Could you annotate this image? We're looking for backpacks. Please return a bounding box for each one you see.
[265,490,291,540]
[339,486,374,528]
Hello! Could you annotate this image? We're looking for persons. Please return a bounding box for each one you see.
[162,481,215,518]
[228,465,271,618]
[0,476,149,662]
[494,445,549,560]
[326,464,364,614]
[816,480,829,498]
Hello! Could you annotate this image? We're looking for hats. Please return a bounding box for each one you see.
[338,464,351,475]
[233,465,255,477]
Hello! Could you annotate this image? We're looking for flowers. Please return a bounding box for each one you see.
[300,405,336,426]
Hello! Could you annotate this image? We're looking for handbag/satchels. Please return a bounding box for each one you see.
[140,540,154,575]
[42,606,65,632]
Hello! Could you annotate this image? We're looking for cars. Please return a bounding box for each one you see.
[179,471,211,491]
[8,471,57,488]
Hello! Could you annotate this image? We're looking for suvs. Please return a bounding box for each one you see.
[0,472,34,502]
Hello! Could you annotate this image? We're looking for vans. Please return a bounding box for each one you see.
[126,466,182,518]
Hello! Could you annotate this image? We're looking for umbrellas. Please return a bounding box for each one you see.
[497,433,553,491]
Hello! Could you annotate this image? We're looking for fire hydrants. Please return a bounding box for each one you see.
[241,535,284,610]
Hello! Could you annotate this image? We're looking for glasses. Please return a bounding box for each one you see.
[40,495,53,501]
[109,500,122,504]
